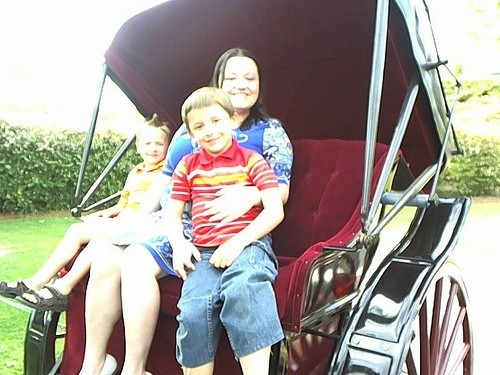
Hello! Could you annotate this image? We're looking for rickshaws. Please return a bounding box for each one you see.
[24,0,476,375]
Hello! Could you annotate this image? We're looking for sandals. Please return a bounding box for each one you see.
[15,285,69,312]
[0,281,30,298]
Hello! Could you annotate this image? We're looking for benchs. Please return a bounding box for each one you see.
[60,140,403,375]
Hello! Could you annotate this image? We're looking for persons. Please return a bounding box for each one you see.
[77,47,293,375]
[159,84,285,375]
[0,122,170,312]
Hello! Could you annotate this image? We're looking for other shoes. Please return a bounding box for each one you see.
[101,354,118,375]
